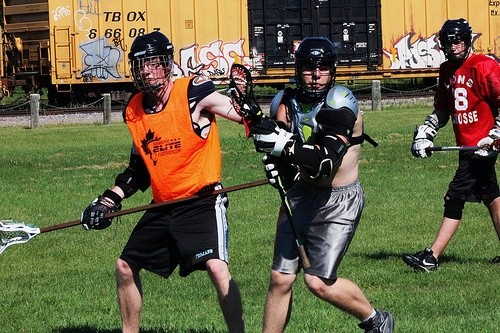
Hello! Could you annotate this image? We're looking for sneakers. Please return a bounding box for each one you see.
[401,246,439,273]
[492,255,500,263]
[358,308,394,333]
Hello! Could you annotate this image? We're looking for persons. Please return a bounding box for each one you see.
[250,37,395,333]
[80,31,245,333]
[402,18,500,274]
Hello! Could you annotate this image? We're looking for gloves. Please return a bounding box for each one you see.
[251,121,300,165]
[411,112,440,158]
[81,188,121,230]
[474,114,500,156]
[261,154,296,189]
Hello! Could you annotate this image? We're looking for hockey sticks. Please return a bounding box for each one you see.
[417,143,500,155]
[0,180,275,257]
[228,63,319,269]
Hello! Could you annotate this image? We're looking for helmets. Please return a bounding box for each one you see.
[128,31,175,91]
[438,16,473,61]
[295,36,338,99]
